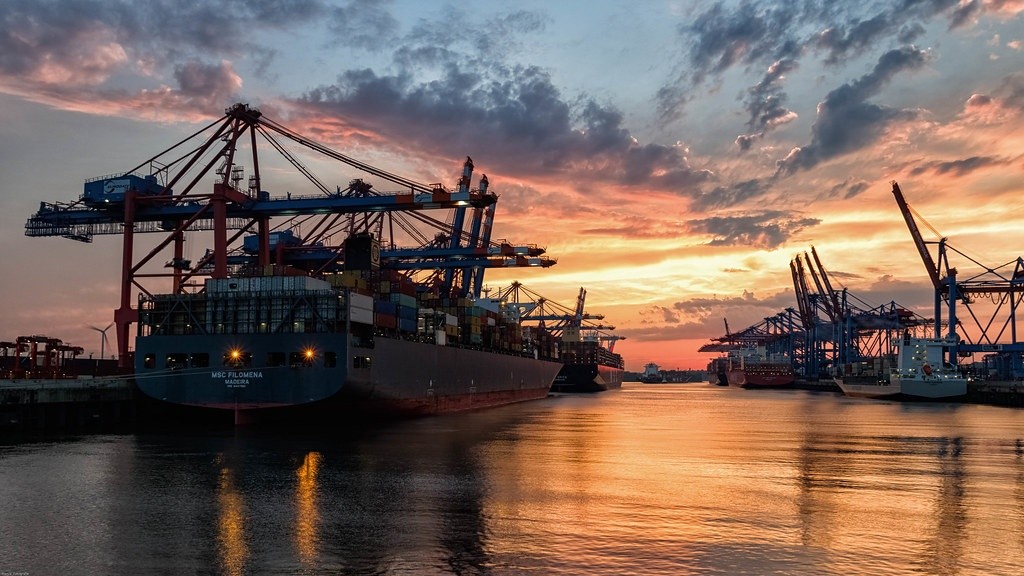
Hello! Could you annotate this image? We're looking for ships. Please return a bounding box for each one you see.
[548,341,625,395]
[129,231,566,432]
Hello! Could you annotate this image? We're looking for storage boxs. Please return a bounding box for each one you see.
[561,341,617,366]
[153,229,560,362]
[83,175,173,208]
[827,355,897,377]
[707,345,791,374]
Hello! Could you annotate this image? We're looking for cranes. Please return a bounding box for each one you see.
[24,102,628,343]
[697,179,1024,385]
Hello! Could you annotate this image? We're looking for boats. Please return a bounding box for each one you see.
[724,340,796,389]
[707,355,730,386]
[832,329,968,403]
[640,363,664,384]
